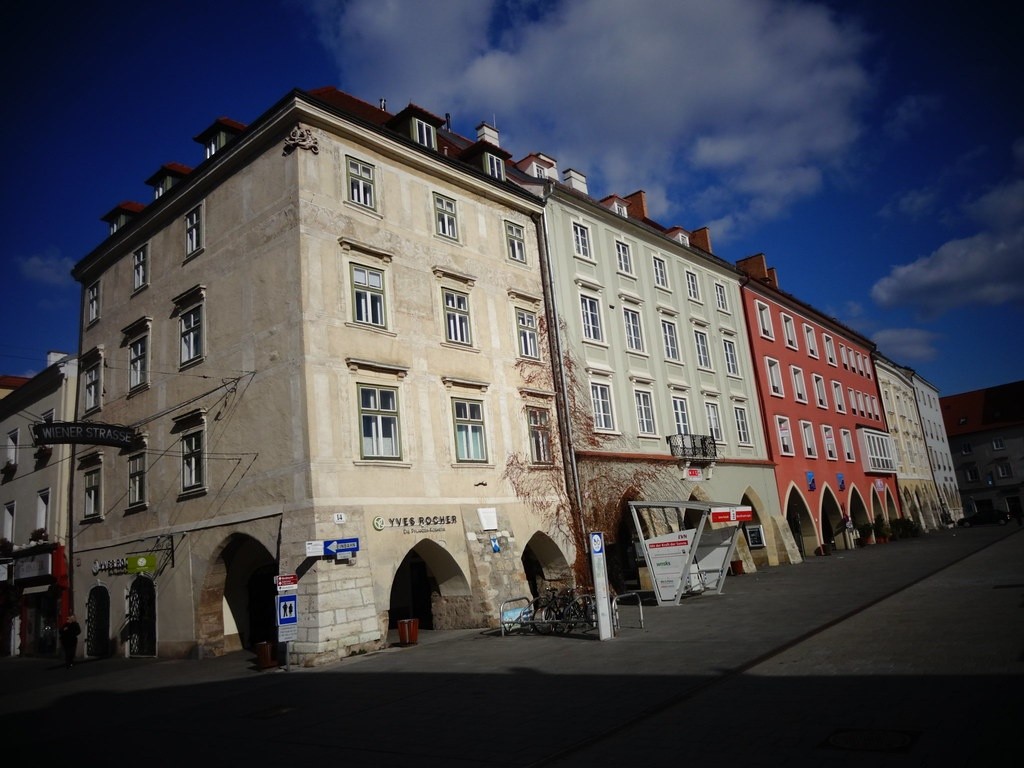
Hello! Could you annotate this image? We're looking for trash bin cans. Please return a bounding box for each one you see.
[257,642,272,668]
[822,544,831,555]
[731,560,742,576]
[400,619,418,646]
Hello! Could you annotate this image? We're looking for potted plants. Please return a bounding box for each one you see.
[858,517,920,544]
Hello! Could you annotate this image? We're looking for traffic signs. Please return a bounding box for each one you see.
[324,537,359,556]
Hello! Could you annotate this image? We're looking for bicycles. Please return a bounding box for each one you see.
[529,587,598,635]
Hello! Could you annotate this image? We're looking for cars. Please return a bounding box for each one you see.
[957,508,1011,527]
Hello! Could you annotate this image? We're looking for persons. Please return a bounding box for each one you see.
[59,614,81,668]
